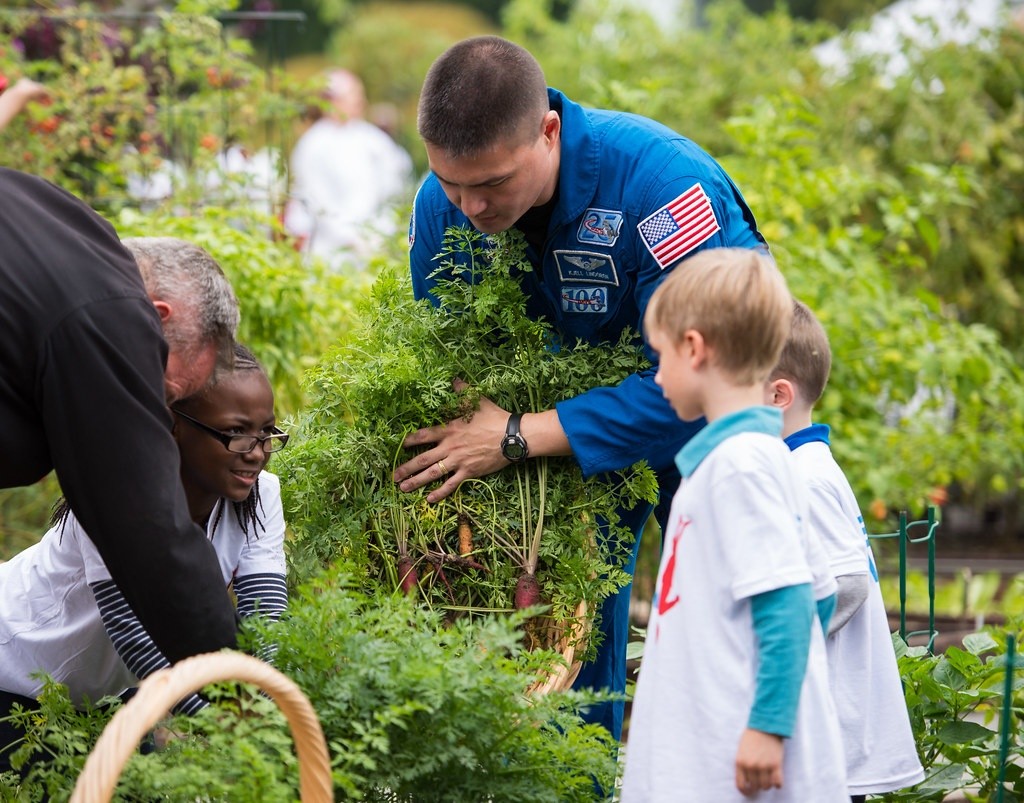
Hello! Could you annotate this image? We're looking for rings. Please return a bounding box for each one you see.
[438,461,449,475]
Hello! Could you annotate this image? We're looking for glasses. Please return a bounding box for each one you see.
[170,407,290,453]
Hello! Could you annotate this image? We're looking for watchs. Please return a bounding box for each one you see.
[500,412,530,463]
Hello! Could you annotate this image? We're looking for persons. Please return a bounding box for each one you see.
[0,341,288,803]
[762,298,926,803]
[392,34,770,802]
[0,76,49,129]
[0,165,255,723]
[122,68,417,286]
[620,247,851,803]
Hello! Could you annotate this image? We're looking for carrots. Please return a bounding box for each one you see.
[515,575,541,651]
[459,523,474,562]
[398,555,436,598]
